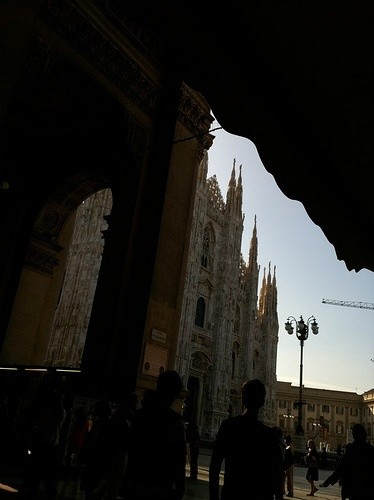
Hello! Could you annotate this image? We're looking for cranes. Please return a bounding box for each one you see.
[321,298,374,310]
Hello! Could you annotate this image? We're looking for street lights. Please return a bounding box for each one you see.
[285,314,320,461]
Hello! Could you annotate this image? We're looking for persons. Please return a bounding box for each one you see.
[271,426,294,500]
[319,423,374,500]
[305,440,319,496]
[208,378,287,500]
[16,370,201,500]
